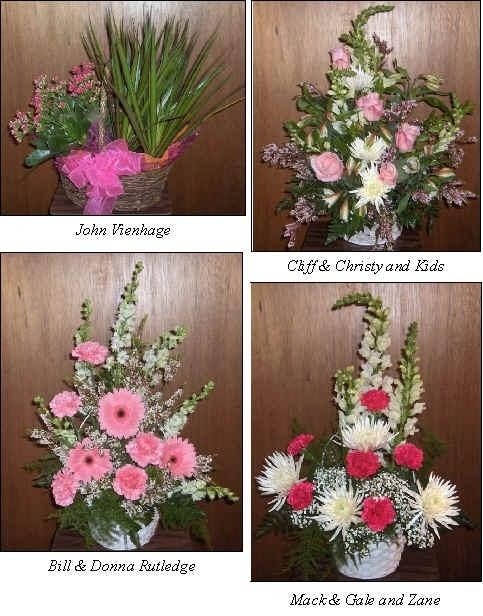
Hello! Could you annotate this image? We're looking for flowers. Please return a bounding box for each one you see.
[22,262,239,552]
[261,4,479,252]
[256,293,475,580]
[6,6,246,167]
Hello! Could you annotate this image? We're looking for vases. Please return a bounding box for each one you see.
[86,505,159,551]
[344,207,402,246]
[329,523,405,580]
[53,157,174,211]
